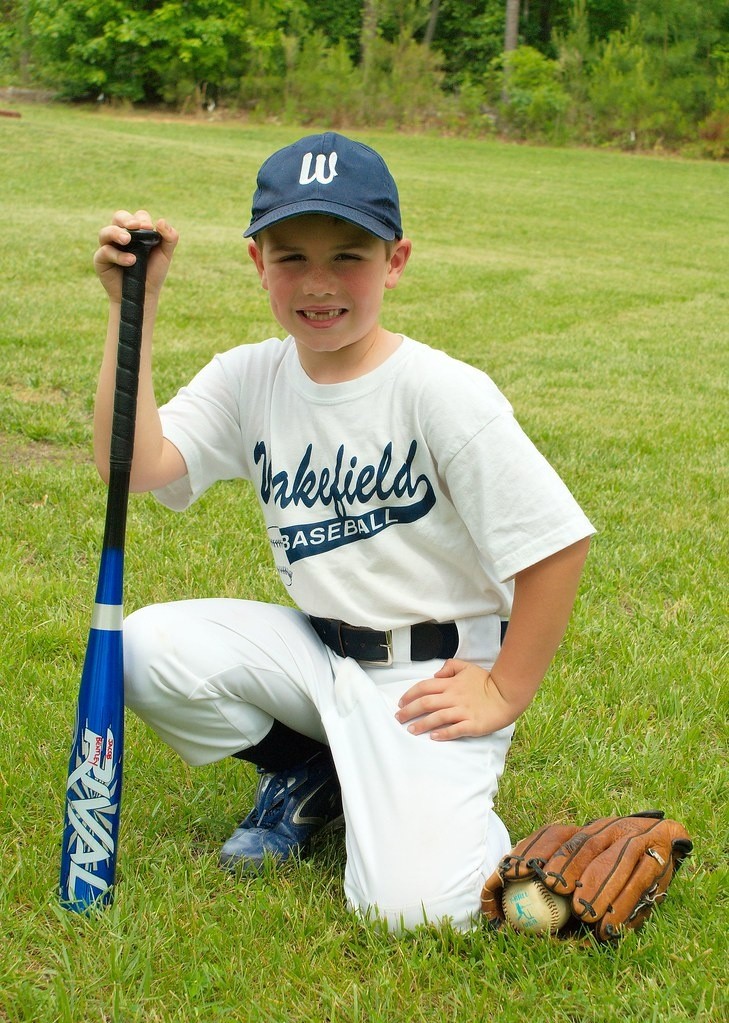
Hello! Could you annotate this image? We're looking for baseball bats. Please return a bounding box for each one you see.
[60,229,166,918]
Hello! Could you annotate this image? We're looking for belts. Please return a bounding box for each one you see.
[309,611,508,662]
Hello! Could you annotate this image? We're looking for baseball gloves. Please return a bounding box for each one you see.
[481,811,693,945]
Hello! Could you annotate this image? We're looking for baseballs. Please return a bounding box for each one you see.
[501,872,569,938]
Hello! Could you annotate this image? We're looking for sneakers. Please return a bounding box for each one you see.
[220,751,343,877]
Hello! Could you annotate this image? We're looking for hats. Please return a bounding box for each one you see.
[241,133,403,242]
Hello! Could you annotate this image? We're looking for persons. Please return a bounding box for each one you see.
[95,133,596,939]
